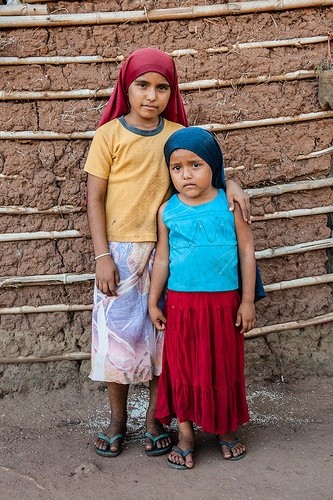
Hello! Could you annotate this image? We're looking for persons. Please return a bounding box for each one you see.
[83,49,253,458]
[147,127,256,470]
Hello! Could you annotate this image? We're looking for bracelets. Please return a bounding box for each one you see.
[93,253,112,261]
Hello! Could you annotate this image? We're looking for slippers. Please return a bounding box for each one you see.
[144,432,174,456]
[93,433,124,457]
[219,438,246,460]
[166,446,197,469]
